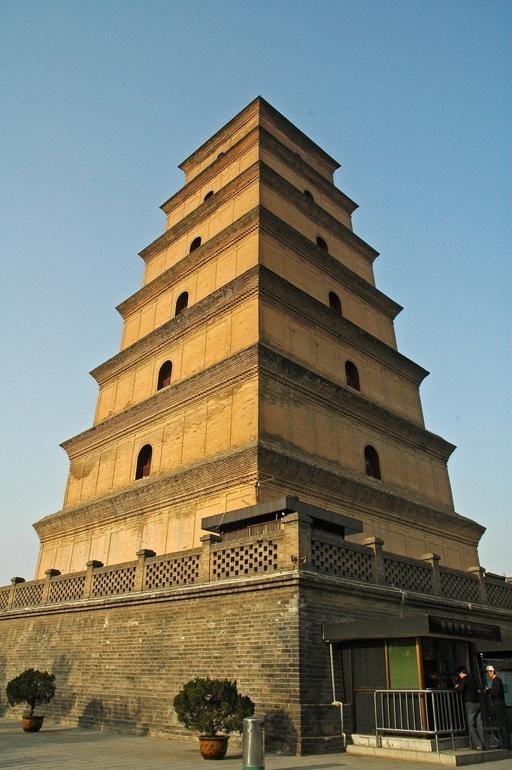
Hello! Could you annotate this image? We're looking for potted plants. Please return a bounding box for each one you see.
[6,668,56,732]
[173,676,255,759]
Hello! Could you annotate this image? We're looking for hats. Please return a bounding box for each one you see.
[485,666,494,671]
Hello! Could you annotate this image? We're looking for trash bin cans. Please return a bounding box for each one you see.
[243,717,264,770]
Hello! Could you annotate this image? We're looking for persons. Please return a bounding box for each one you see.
[482,664,510,751]
[454,666,487,752]
